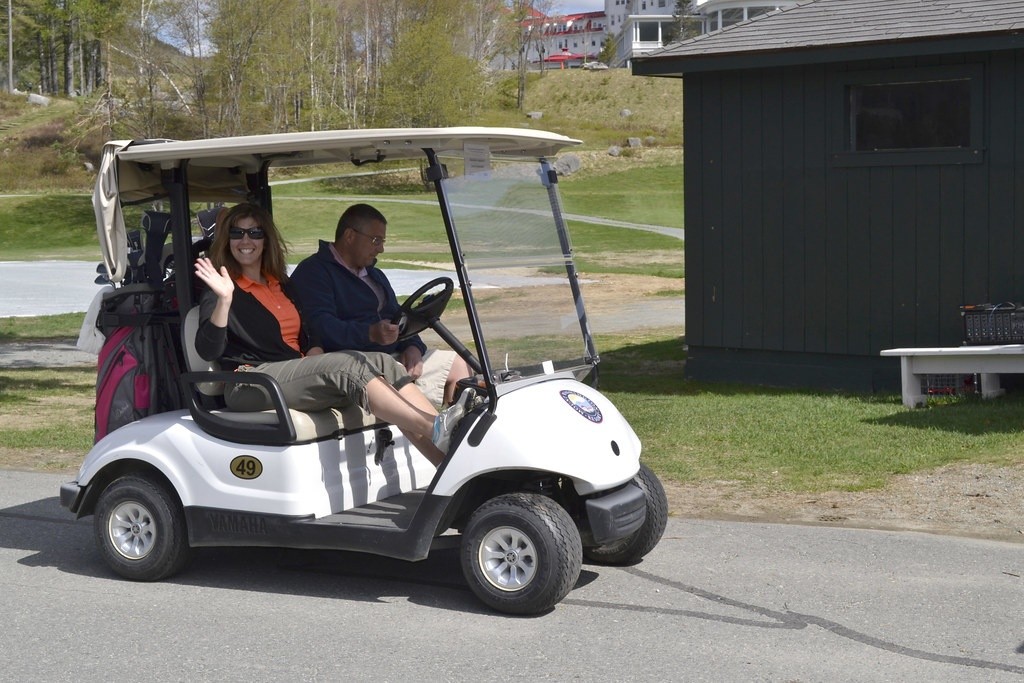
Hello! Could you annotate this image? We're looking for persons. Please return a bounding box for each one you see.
[194,201,478,472]
[290,202,476,407]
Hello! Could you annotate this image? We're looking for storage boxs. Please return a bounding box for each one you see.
[919,374,975,396]
[960,303,1024,345]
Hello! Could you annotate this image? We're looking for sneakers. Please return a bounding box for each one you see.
[434,388,476,455]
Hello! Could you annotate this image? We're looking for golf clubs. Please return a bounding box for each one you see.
[93,195,233,291]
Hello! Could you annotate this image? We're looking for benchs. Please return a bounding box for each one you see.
[880,344,1023,408]
[181,306,390,443]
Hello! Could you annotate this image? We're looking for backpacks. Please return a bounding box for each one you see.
[94,322,156,445]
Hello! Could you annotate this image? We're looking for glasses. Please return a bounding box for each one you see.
[358,231,381,245]
[231,225,264,239]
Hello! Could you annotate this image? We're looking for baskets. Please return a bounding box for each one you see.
[962,303,1024,345]
[922,374,977,400]
